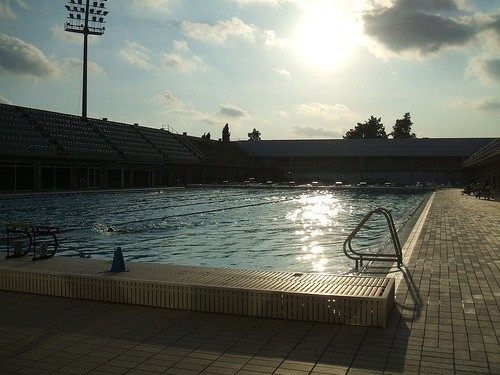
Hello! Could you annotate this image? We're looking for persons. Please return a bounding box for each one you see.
[107,228,135,233]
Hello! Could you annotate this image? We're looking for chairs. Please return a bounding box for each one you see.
[0,103,253,163]
[416,138,499,199]
